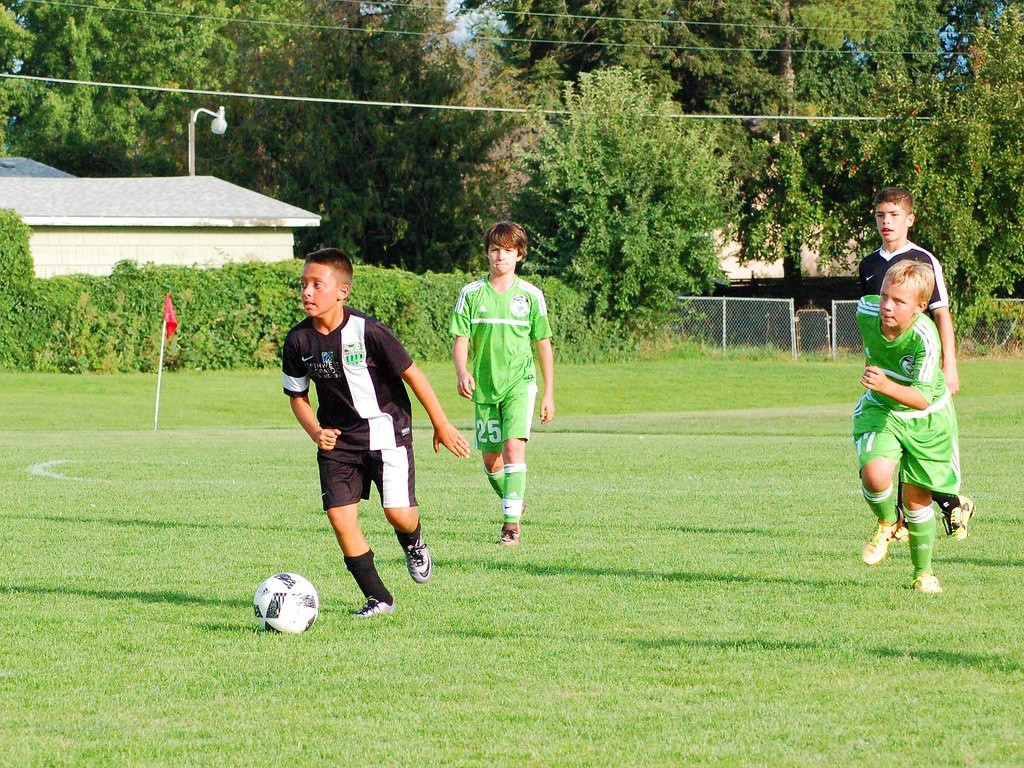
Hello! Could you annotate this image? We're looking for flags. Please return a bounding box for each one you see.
[163,293,178,339]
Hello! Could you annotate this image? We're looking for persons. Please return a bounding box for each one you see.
[448,220,556,546]
[858,188,977,542]
[852,259,960,595]
[281,247,471,618]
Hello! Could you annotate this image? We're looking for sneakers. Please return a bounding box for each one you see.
[862,504,903,564]
[911,571,942,593]
[940,495,976,541]
[519,502,527,521]
[353,594,396,618]
[499,522,521,546]
[404,535,431,583]
[889,516,910,542]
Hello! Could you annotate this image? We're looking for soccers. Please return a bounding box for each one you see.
[254,572,318,635]
